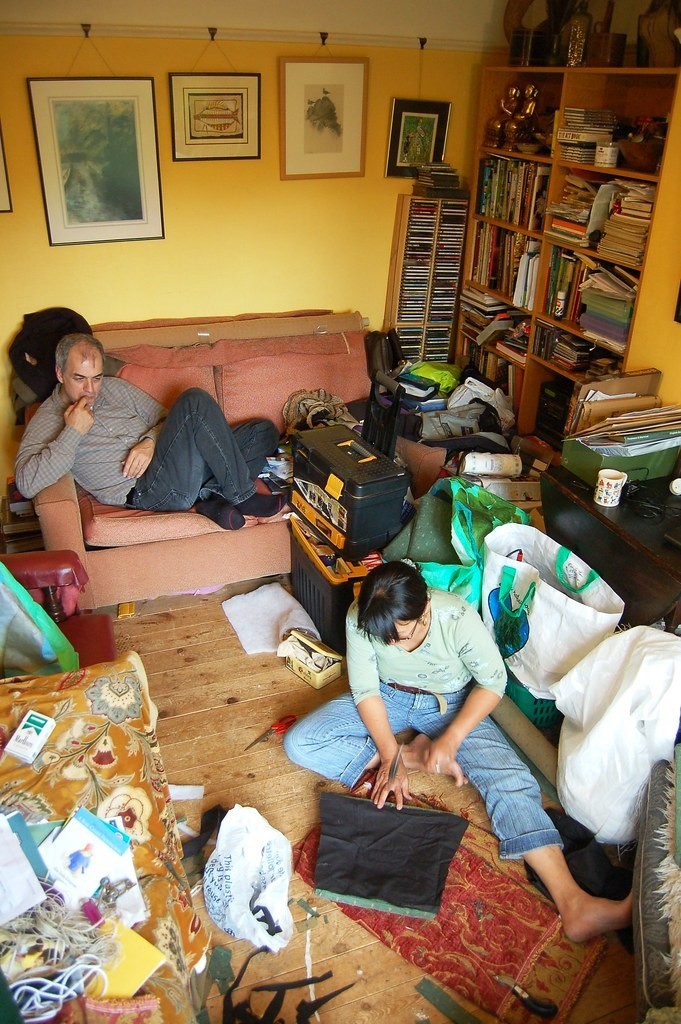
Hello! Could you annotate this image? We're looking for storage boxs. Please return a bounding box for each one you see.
[444,435,555,536]
[5,709,56,767]
[284,630,343,690]
[558,367,681,487]
[558,131,614,142]
[287,514,369,585]
[285,424,409,564]
[283,525,360,656]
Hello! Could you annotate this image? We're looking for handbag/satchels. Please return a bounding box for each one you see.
[448,377,516,431]
[202,804,297,952]
[420,403,485,440]
[482,523,626,702]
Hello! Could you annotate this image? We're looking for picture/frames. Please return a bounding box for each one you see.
[0,126,13,214]
[383,97,452,179]
[27,77,165,247]
[168,71,262,162]
[278,55,369,183]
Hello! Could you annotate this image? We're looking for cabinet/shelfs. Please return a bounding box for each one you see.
[382,195,469,362]
[456,64,681,467]
[540,458,681,630]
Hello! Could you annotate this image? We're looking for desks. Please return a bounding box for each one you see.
[0,653,200,1024]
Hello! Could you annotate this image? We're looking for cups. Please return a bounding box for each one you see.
[593,468,628,508]
[593,140,619,168]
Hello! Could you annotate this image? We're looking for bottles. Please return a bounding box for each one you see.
[554,290,566,321]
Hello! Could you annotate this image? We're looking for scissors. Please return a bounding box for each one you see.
[244,713,299,751]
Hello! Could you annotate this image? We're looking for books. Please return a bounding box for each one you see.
[261,448,293,495]
[0,475,45,554]
[459,108,681,446]
[0,805,131,927]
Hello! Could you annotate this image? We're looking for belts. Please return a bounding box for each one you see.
[127,488,136,510]
[388,682,448,715]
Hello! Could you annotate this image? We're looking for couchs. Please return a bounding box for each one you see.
[24,310,446,614]
[0,550,116,673]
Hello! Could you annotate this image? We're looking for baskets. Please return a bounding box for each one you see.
[504,672,563,727]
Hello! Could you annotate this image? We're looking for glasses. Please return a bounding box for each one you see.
[364,619,419,641]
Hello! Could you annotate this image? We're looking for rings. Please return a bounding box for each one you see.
[138,464,143,466]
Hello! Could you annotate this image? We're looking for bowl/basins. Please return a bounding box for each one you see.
[618,138,664,172]
[516,144,544,155]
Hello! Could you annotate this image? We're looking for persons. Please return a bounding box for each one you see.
[283,556,633,943]
[13,332,287,531]
